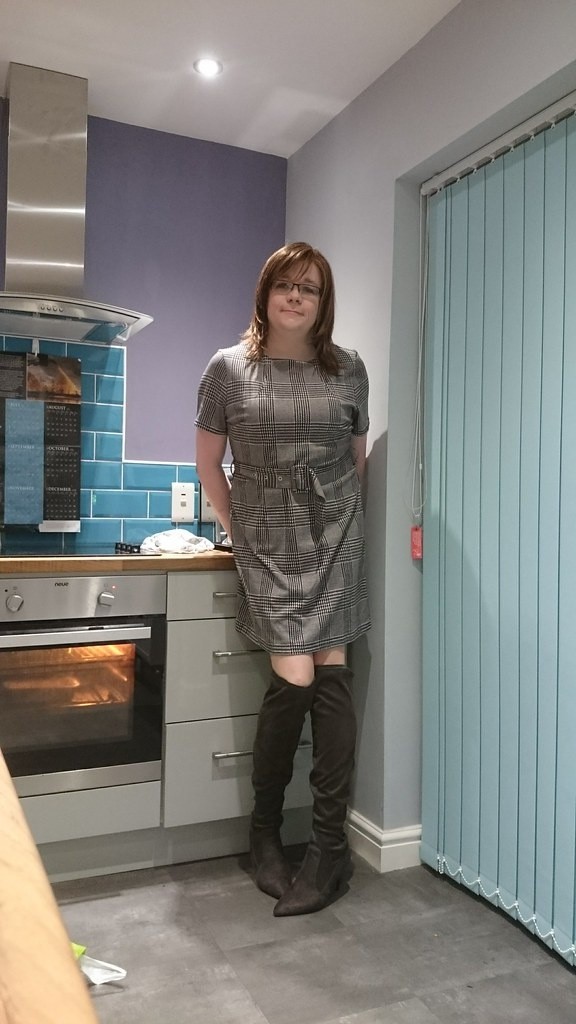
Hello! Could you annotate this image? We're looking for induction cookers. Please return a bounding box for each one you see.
[0,540,161,557]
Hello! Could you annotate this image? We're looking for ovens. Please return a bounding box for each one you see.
[0,578,164,792]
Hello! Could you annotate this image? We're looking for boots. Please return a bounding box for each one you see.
[247,666,314,898]
[272,664,357,917]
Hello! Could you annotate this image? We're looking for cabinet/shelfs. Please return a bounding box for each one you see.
[162,571,315,828]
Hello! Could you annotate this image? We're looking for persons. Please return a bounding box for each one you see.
[194,242,369,917]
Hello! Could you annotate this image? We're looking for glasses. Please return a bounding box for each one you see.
[273,279,323,298]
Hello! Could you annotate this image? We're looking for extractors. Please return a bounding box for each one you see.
[0,61,155,350]
